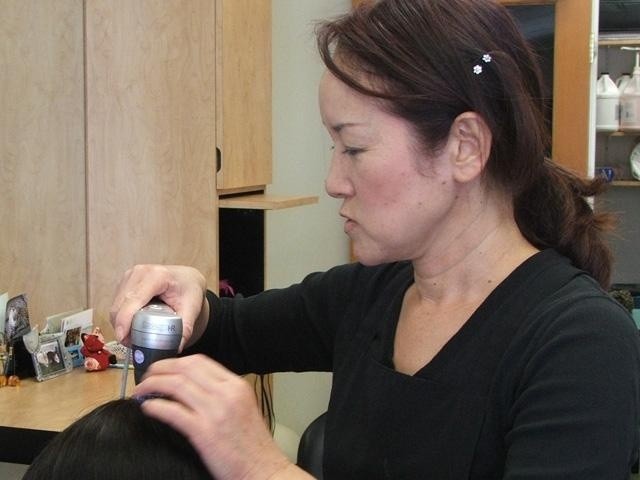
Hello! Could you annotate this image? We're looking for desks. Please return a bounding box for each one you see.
[1,362,143,447]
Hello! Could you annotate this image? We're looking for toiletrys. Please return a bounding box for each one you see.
[619,45,640,134]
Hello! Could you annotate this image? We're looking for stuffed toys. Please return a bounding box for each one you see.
[82,334,116,370]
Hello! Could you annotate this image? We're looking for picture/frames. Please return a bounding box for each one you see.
[32,337,73,383]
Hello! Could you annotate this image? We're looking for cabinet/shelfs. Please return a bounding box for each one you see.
[216,0,272,195]
[0,1,220,356]
[550,0,639,210]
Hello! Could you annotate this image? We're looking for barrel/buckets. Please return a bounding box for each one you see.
[595,72,620,133]
[615,72,631,92]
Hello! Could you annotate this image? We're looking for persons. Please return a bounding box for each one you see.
[23,398,212,480]
[110,1,639,480]
